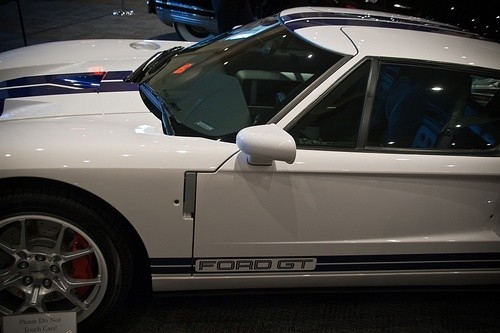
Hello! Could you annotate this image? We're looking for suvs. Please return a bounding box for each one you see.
[0,7,499,330]
